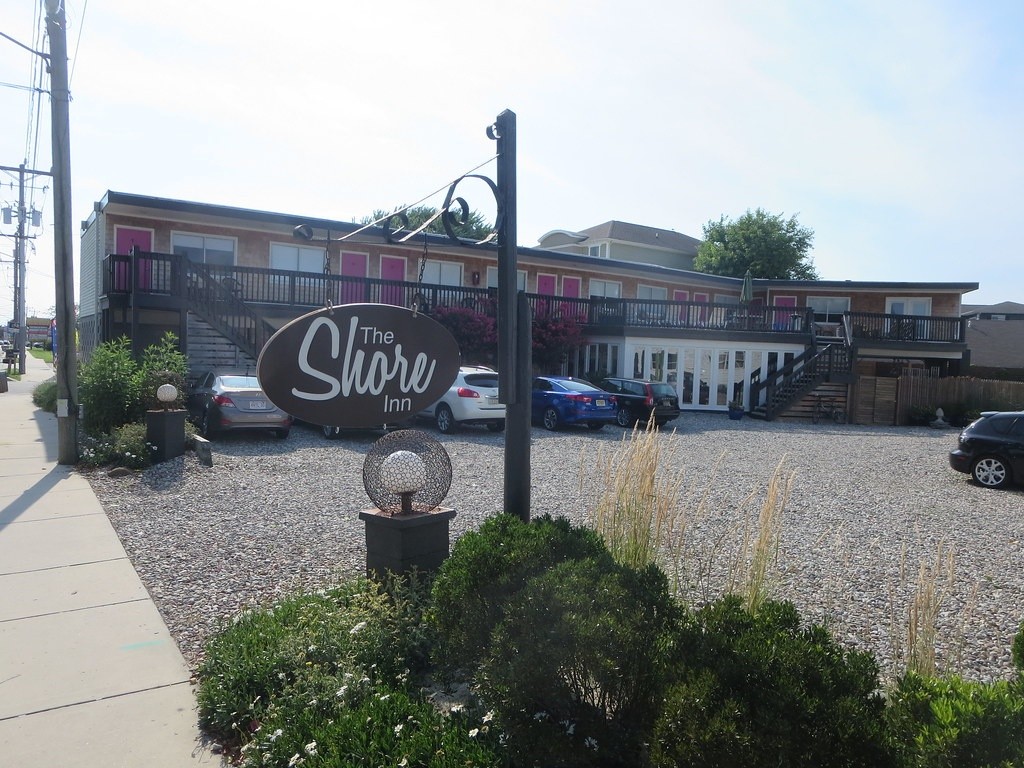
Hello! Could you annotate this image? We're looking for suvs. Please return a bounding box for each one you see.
[414,365,503,435]
[596,378,680,428]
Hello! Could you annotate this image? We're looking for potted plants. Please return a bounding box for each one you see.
[727,400,745,420]
[947,400,985,426]
[912,404,938,426]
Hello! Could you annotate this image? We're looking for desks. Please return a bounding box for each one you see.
[437,306,448,313]
[730,315,764,331]
[197,288,216,301]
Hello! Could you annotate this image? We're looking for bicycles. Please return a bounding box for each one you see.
[812,395,846,425]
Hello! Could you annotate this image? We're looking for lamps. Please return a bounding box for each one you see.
[363,431,454,516]
[157,384,177,411]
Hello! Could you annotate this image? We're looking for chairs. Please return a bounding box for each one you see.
[415,292,432,314]
[814,322,822,336]
[178,275,198,297]
[461,298,477,314]
[635,305,729,328]
[219,277,244,303]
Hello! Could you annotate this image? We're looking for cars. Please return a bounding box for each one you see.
[188,367,291,439]
[531,375,617,431]
[949,412,1024,488]
